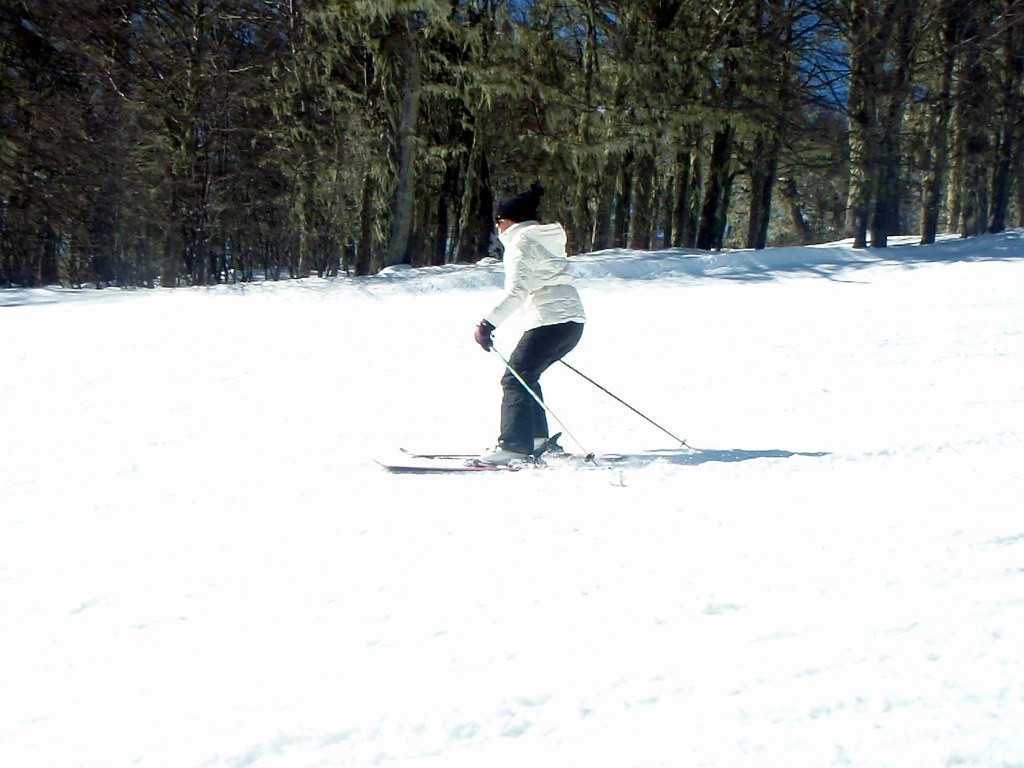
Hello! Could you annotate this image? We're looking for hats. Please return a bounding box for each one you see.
[496,183,545,223]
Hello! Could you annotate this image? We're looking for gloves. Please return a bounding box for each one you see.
[474,319,496,353]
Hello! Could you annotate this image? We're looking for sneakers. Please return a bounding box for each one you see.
[478,446,530,466]
[533,438,549,451]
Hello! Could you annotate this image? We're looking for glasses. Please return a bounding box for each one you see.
[495,217,503,226]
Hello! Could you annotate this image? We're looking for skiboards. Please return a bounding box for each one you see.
[371,443,628,472]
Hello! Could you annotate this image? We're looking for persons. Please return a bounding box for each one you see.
[473,182,590,465]
